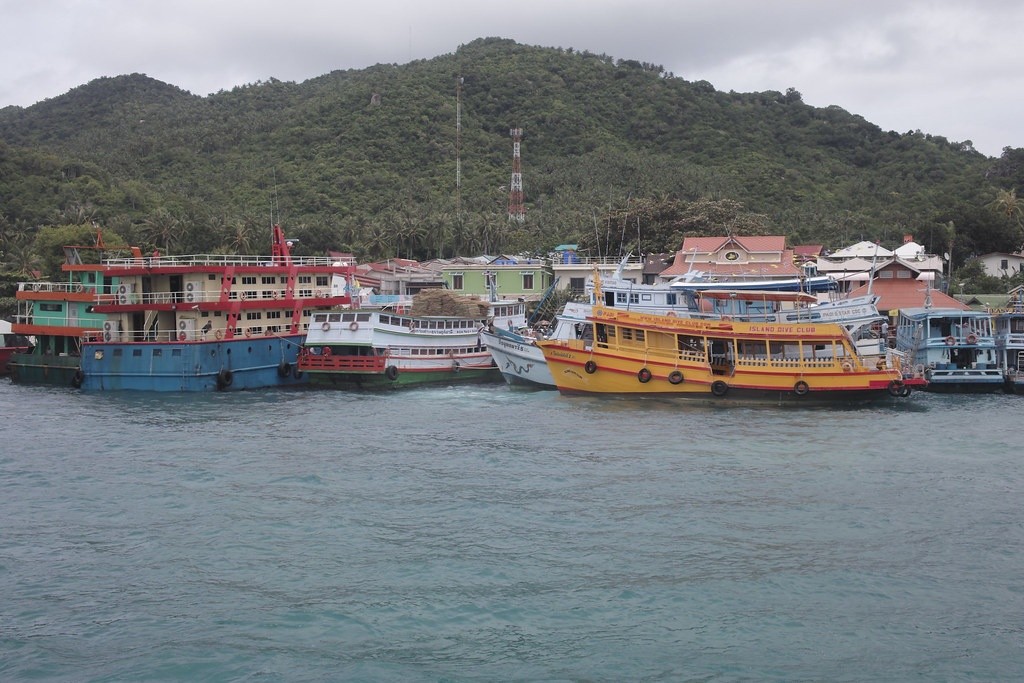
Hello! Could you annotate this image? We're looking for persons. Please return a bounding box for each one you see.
[508,320,513,333]
[200,320,212,340]
[881,319,890,343]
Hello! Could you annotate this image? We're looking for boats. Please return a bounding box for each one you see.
[3,214,1022,407]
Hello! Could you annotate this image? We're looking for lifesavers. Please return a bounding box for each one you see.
[667,312,676,318]
[216,329,222,339]
[246,328,250,336]
[240,292,246,299]
[350,322,359,331]
[409,322,415,332]
[315,289,321,297]
[273,290,278,300]
[508,320,512,326]
[76,285,83,293]
[32,284,39,292]
[945,336,955,345]
[321,323,331,332]
[722,315,731,322]
[967,334,976,345]
